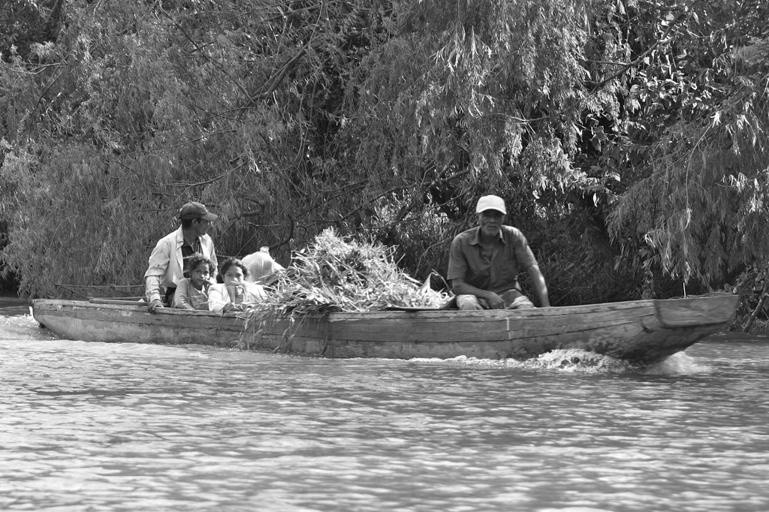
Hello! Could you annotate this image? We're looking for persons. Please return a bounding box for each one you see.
[172,250,213,311]
[143,200,219,314]
[446,193,552,310]
[206,256,269,315]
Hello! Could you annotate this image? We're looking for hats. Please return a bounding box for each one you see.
[474,194,508,216]
[179,201,218,222]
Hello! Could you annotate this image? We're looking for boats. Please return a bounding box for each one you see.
[31,293,740,359]
